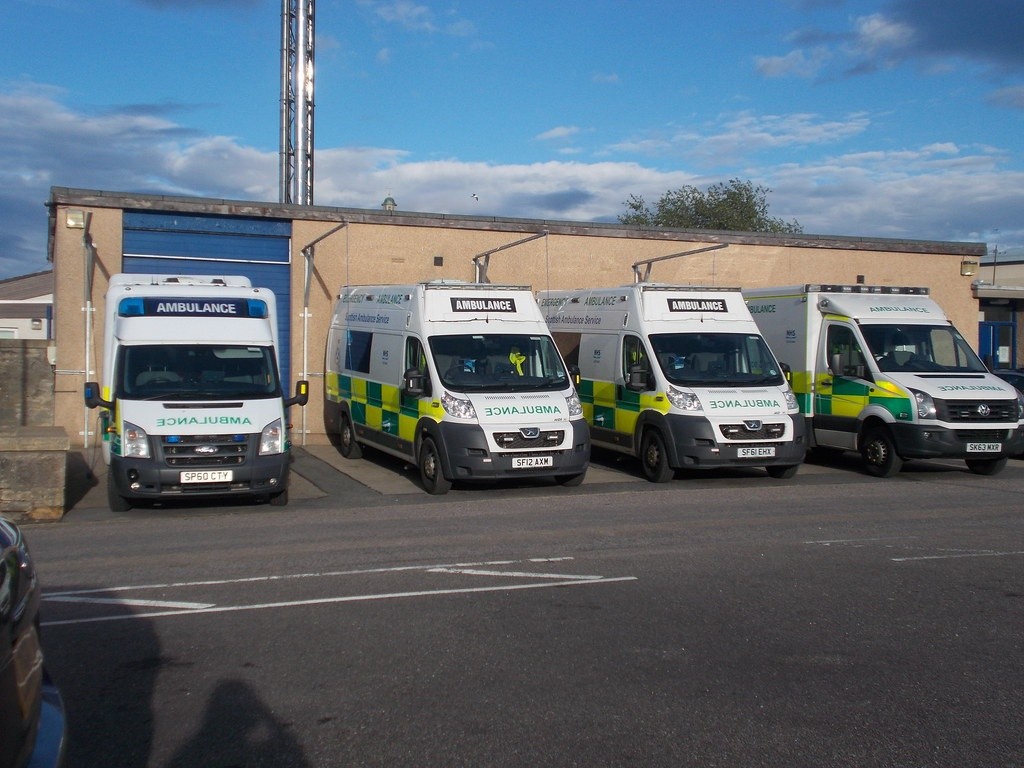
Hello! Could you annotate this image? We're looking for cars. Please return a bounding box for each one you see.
[0,506,47,768]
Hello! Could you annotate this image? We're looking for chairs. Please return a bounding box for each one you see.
[835,329,868,376]
[424,341,459,396]
[649,340,681,372]
[202,358,252,393]
[685,336,724,374]
[136,348,193,396]
[888,330,914,366]
[485,338,512,375]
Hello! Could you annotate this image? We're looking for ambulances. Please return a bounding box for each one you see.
[322,281,594,495]
[85,272,309,510]
[532,275,812,486]
[748,282,1024,491]
